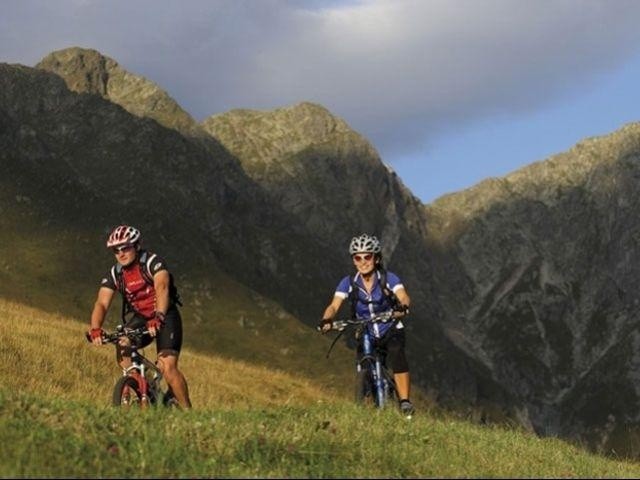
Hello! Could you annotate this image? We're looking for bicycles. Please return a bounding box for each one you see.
[85,318,184,410]
[318,307,410,411]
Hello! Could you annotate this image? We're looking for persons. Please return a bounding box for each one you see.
[315,231,414,417]
[89,223,191,415]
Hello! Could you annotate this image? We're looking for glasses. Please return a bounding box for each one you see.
[353,253,373,261]
[113,245,135,254]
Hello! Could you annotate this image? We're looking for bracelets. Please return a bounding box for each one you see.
[150,311,165,324]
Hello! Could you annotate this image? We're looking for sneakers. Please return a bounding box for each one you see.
[400,400,415,415]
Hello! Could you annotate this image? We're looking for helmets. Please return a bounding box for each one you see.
[349,233,381,259]
[106,224,142,250]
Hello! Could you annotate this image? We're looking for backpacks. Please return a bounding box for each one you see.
[115,251,183,324]
